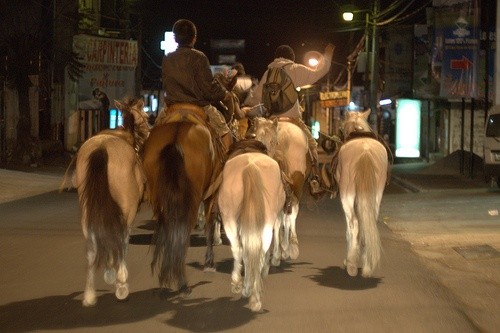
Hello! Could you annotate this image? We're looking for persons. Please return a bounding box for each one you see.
[253,43,336,194]
[161,19,253,154]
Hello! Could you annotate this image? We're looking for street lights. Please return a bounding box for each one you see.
[342,0,416,138]
[302,58,320,132]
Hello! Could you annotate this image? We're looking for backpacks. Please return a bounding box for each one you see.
[258,60,300,114]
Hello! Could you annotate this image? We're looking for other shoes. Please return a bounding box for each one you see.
[310,178,320,195]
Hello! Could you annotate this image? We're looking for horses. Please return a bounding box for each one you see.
[59,94,394,314]
